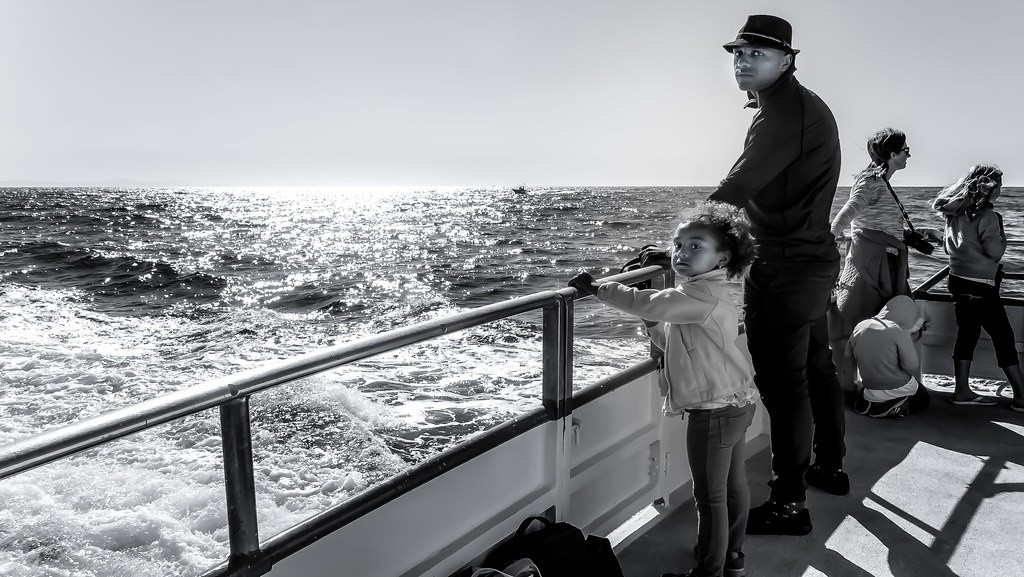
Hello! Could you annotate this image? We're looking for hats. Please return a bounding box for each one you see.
[723,14,800,56]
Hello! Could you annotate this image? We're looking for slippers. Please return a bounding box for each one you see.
[948,394,998,406]
[1011,404,1024,412]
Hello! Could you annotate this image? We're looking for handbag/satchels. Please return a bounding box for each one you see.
[482,516,625,577]
[471,558,542,577]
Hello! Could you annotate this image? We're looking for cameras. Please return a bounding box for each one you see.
[903,230,935,255]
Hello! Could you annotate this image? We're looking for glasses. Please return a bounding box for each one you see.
[900,147,910,152]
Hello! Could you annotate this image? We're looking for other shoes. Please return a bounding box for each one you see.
[745,500,813,536]
[661,568,695,577]
[868,396,910,418]
[693,544,746,572]
[803,463,850,496]
[854,387,871,414]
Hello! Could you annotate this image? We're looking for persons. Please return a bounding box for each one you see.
[844,295,932,418]
[831,128,944,333]
[638,14,851,536]
[567,202,760,577]
[932,165,1024,412]
